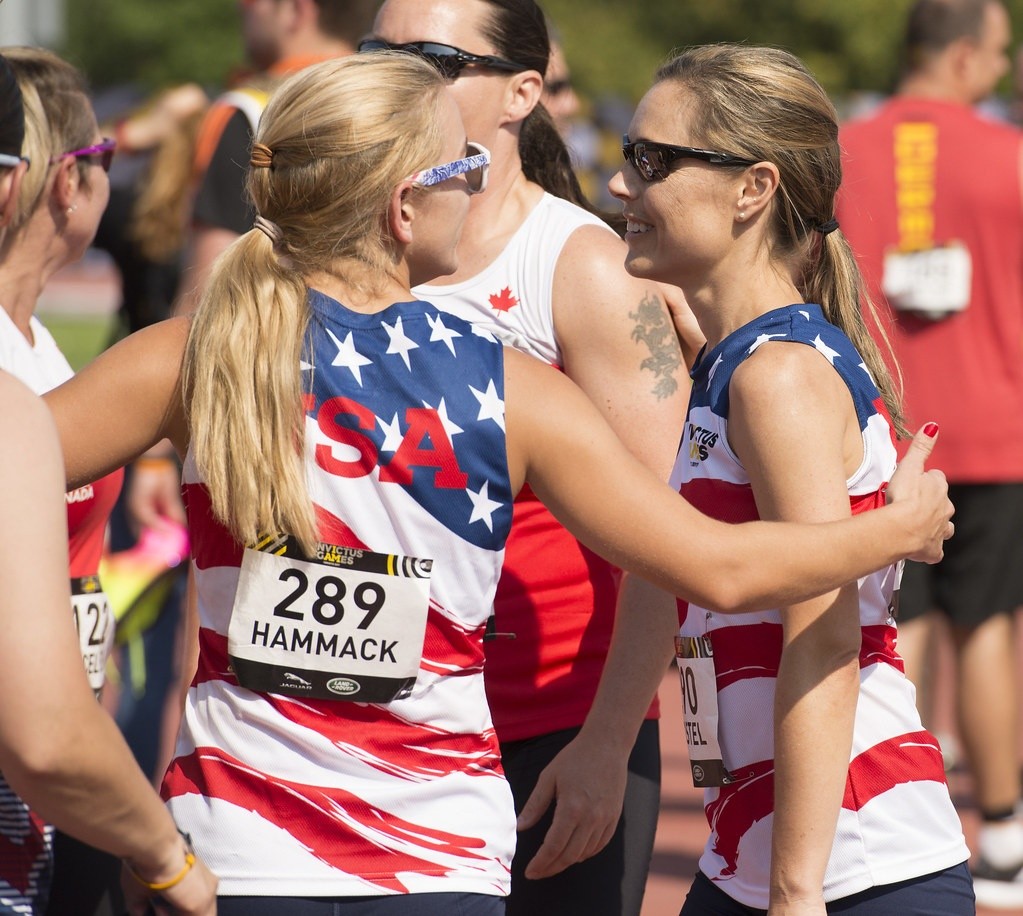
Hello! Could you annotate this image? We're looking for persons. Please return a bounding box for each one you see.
[831,0,1023,880]
[39,52,956,916]
[610,47,977,915]
[0,0,690,916]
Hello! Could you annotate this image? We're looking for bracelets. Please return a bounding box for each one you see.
[122,829,195,890]
[134,456,173,468]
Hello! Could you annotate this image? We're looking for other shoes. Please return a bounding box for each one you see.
[970,806,1023,910]
[935,731,977,802]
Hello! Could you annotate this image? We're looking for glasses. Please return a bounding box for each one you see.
[544,80,567,94]
[51,136,116,172]
[358,39,529,80]
[622,133,761,182]
[404,141,491,194]
